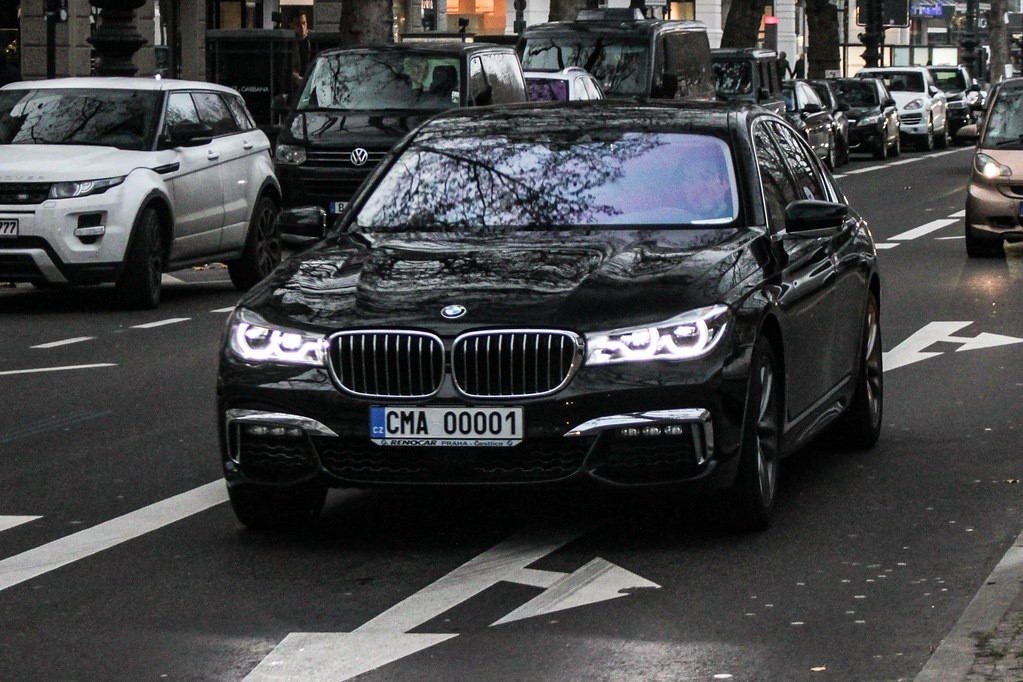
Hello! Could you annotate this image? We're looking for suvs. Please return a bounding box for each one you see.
[709,46,786,122]
[853,67,951,149]
[518,66,608,105]
[923,64,983,132]
[0,76,283,310]
[217,102,882,536]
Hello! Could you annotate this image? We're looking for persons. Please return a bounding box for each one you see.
[676,153,733,219]
[282,12,318,93]
[777,51,792,81]
[791,54,805,79]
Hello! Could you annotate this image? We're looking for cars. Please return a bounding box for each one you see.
[784,79,851,169]
[966,76,1023,260]
[832,79,903,159]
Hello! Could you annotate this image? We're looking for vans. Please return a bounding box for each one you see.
[271,37,532,226]
[516,8,715,106]
